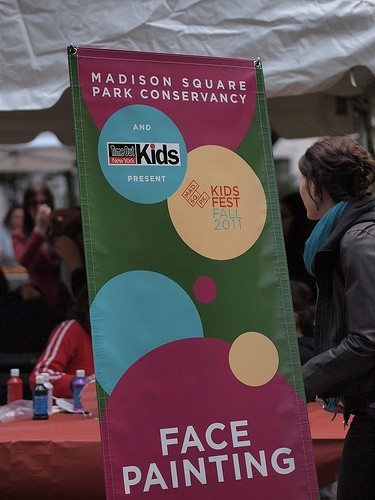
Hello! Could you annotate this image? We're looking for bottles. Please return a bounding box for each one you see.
[73,369,87,412]
[32,376,49,420]
[41,373,54,416]
[7,368,23,404]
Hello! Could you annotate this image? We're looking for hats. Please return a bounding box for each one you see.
[49,207,81,237]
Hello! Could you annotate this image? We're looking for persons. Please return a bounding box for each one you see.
[280,192,320,366]
[299,136,375,500]
[0,183,95,399]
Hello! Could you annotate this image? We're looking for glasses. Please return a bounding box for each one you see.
[30,201,49,208]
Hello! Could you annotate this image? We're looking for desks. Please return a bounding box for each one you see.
[0,397,354,500]
